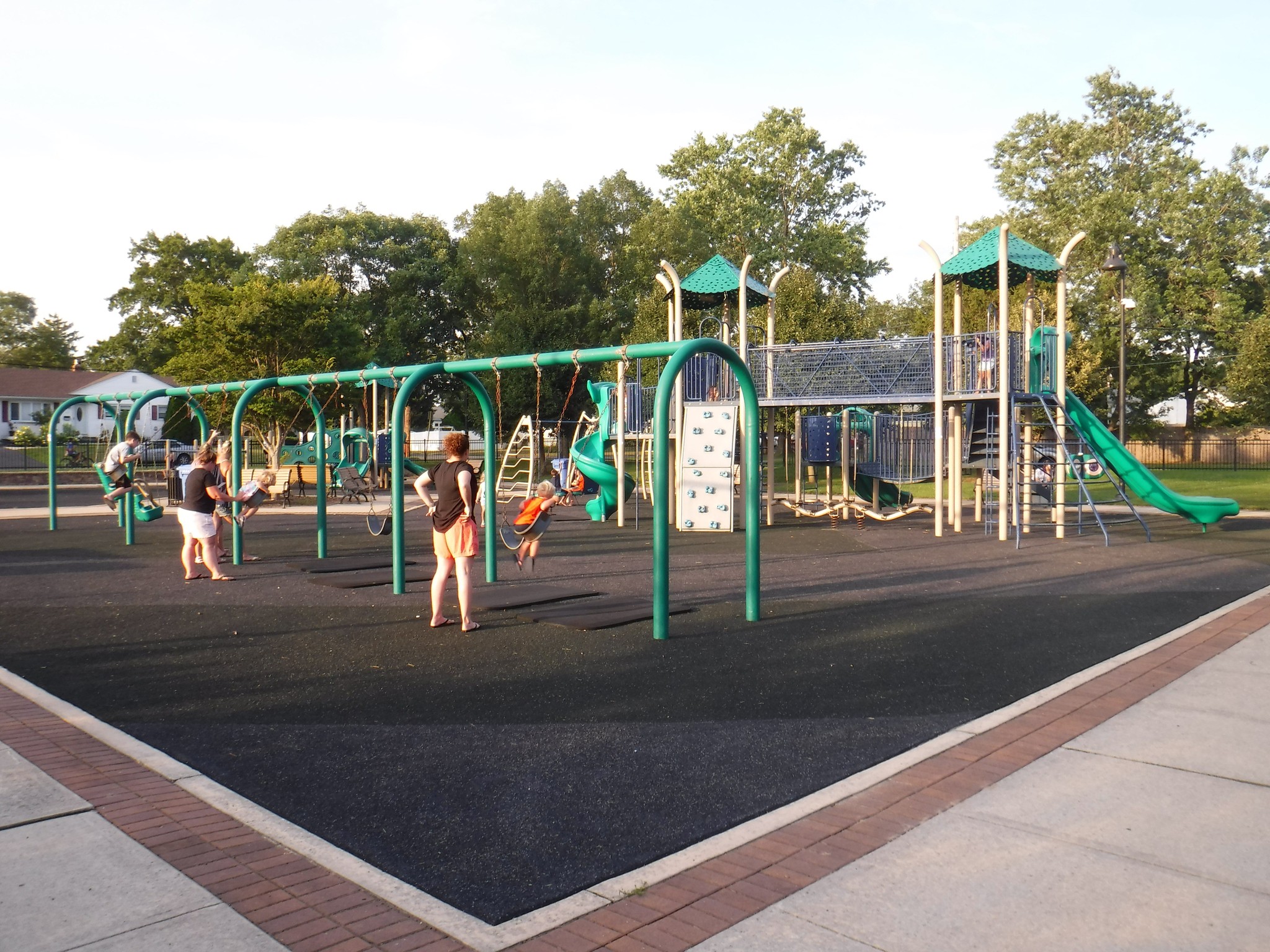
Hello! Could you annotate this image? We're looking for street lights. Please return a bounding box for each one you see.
[1101,242,1128,500]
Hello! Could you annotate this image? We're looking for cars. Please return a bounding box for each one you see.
[132,439,200,464]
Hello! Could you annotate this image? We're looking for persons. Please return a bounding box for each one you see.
[512,480,560,573]
[162,452,175,480]
[233,472,276,528]
[1031,459,1056,492]
[177,430,262,580]
[568,468,584,506]
[414,432,480,632]
[104,431,142,512]
[65,437,81,465]
[476,470,485,527]
[708,386,720,402]
[970,331,996,393]
[550,461,570,507]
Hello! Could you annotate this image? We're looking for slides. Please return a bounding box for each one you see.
[325,456,372,488]
[404,457,435,484]
[1040,384,1240,523]
[840,460,914,509]
[569,417,636,523]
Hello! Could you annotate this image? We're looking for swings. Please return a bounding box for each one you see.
[513,363,632,544]
[494,369,542,551]
[129,400,164,522]
[362,381,399,537]
[107,394,214,482]
[92,402,126,502]
[241,385,341,509]
[206,389,247,450]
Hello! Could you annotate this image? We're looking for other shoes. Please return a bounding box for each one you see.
[481,522,486,528]
[223,515,233,524]
[234,515,246,528]
[512,553,523,572]
[525,556,535,572]
[102,496,116,511]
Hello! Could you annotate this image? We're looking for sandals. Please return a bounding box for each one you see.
[195,556,204,563]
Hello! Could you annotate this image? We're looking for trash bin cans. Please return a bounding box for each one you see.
[168,470,183,508]
[551,459,599,494]
[176,464,191,501]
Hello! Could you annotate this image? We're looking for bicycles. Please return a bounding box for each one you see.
[59,450,94,469]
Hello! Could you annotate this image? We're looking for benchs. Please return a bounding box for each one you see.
[973,467,1055,506]
[734,464,741,495]
[466,457,485,480]
[242,468,294,509]
[267,463,338,498]
[336,466,379,503]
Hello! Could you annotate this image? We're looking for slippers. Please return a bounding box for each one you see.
[219,553,233,558]
[213,575,236,581]
[430,618,455,628]
[243,556,261,561]
[184,574,210,580]
[219,559,226,564]
[461,622,481,632]
[224,548,230,552]
